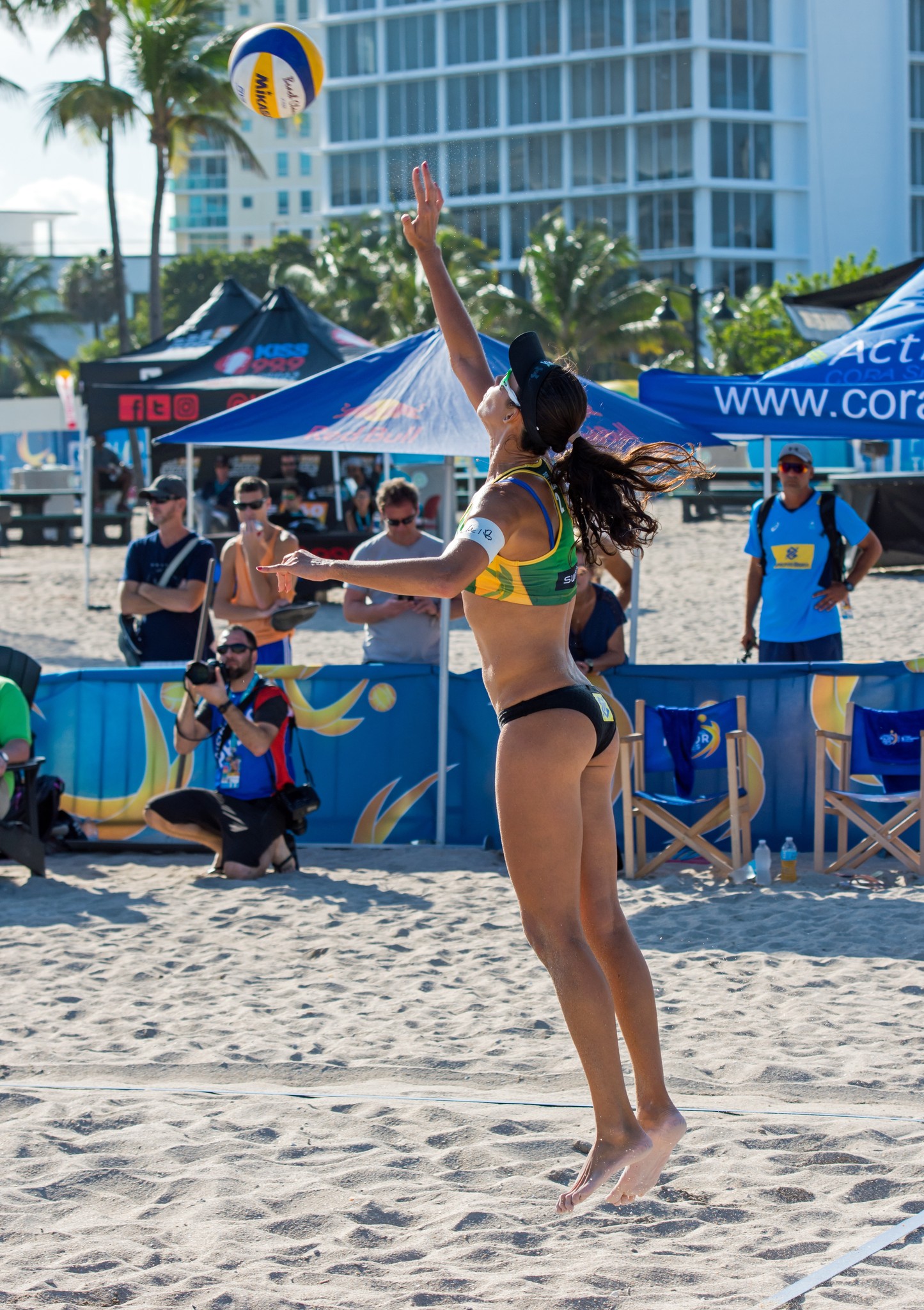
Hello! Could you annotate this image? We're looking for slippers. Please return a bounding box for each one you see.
[270,833,299,874]
[208,866,226,875]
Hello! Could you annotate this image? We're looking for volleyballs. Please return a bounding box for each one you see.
[227,21,325,120]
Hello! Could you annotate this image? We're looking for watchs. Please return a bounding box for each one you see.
[216,700,233,714]
[843,579,853,591]
[0,751,9,762]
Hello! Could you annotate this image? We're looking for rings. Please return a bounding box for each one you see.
[828,600,833,604]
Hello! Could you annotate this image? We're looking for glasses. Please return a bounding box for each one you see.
[385,514,416,526]
[779,462,810,474]
[147,496,167,504]
[217,643,252,656]
[234,497,267,511]
[499,368,539,430]
[282,461,296,466]
[282,494,296,500]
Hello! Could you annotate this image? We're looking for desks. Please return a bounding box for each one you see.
[686,468,856,521]
[0,489,84,546]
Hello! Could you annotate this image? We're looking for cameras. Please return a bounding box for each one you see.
[278,781,321,838]
[186,658,230,685]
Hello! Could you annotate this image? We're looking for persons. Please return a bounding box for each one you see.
[213,478,298,667]
[251,160,716,1214]
[139,626,323,880]
[344,476,453,667]
[742,444,886,664]
[118,474,218,663]
[0,664,43,837]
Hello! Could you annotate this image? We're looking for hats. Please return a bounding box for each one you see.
[779,443,812,463]
[508,332,550,448]
[138,474,186,501]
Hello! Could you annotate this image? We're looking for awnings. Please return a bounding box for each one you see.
[628,259,924,664]
[152,327,758,665]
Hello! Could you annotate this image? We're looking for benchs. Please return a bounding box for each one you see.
[671,491,765,522]
[0,513,132,547]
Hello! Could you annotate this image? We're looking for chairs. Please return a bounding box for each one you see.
[814,702,924,876]
[618,696,752,882]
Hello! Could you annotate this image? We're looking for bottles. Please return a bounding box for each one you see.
[728,858,772,884]
[781,836,797,882]
[755,840,771,886]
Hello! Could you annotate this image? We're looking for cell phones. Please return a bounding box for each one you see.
[398,595,415,600]
